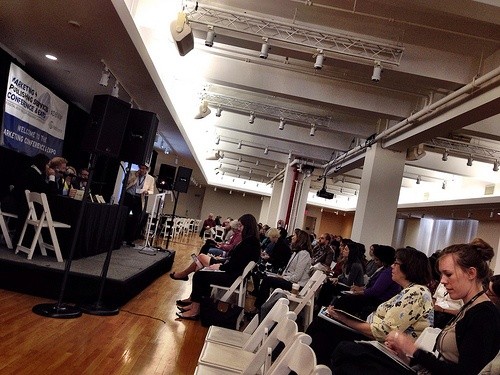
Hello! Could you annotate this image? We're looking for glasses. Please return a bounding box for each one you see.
[321,237,325,239]
[394,261,401,266]
[239,224,243,227]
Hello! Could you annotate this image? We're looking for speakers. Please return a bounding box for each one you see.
[406,146,426,161]
[115,106,160,166]
[195,105,211,119]
[207,152,220,159]
[170,19,194,57]
[174,166,193,193]
[86,93,132,154]
[156,163,176,191]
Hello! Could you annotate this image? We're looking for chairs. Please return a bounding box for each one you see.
[146,215,335,374]
[16,189,70,264]
[0,208,17,249]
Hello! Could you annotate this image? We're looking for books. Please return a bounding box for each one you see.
[318,306,364,331]
[355,339,417,375]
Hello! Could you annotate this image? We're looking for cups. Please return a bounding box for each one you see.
[291,284,300,294]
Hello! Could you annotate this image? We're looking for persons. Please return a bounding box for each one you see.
[122,165,155,247]
[5,153,89,216]
[171,213,500,375]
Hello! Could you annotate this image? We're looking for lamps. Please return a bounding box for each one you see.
[370,61,382,81]
[492,159,500,172]
[406,143,428,162]
[450,205,500,219]
[397,210,428,219]
[416,174,422,184]
[258,38,272,59]
[169,11,199,56]
[311,49,327,70]
[175,98,359,217]
[442,180,447,190]
[442,150,451,161]
[467,154,474,166]
[204,23,216,47]
[96,65,170,155]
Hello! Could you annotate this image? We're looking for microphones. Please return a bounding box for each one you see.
[65,169,85,179]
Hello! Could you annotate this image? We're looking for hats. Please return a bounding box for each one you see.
[142,163,149,168]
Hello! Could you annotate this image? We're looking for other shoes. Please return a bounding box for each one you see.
[170,272,188,281]
[126,241,135,247]
[176,313,200,321]
[176,300,192,306]
[177,307,187,312]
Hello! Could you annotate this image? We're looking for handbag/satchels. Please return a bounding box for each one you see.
[209,248,223,257]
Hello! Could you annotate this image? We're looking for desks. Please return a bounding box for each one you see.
[28,189,131,261]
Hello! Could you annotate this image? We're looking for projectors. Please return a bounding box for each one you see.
[317,190,334,199]
